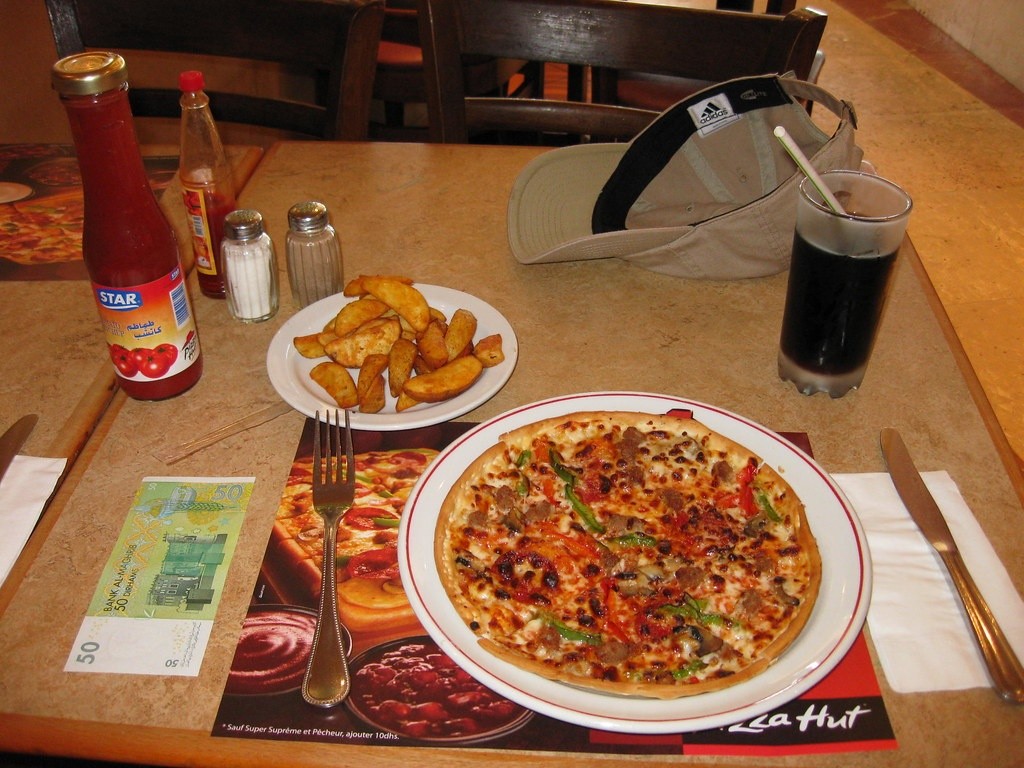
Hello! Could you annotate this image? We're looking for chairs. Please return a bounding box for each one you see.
[43,0,830,145]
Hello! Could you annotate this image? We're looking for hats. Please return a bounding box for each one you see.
[506,70,865,281]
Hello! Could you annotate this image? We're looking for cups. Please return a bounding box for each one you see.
[777,170,913,400]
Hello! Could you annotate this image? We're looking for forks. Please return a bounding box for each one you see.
[302,404,355,709]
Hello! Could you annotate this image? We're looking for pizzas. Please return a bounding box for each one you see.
[0,192,87,266]
[435,408,823,699]
[273,447,446,592]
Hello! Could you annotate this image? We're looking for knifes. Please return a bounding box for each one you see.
[879,427,1024,703]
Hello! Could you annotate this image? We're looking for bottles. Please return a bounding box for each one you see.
[50,51,203,402]
[220,208,280,324]
[177,70,237,300]
[286,201,343,309]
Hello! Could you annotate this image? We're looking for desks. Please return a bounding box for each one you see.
[0,133,1024,768]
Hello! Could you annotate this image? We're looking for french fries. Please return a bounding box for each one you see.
[291,271,505,412]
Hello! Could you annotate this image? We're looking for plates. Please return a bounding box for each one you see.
[265,284,518,431]
[397,390,873,735]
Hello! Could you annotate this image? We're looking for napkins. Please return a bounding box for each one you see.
[0,454,67,588]
[829,470,1024,695]
